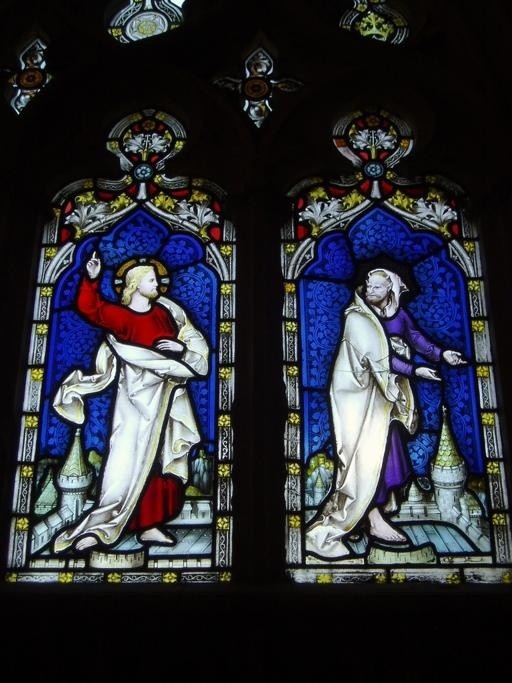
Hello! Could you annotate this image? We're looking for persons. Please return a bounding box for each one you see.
[305,266,469,557]
[49,246,213,552]
